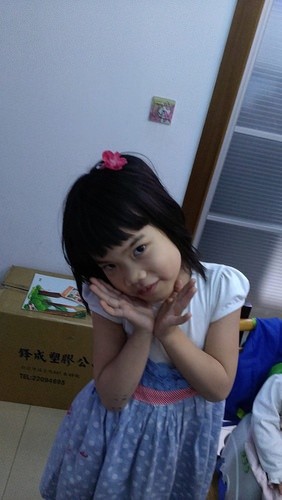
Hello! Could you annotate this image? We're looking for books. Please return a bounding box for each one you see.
[21,273,86,321]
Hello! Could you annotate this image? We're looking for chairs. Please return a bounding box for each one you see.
[207,318,282,500]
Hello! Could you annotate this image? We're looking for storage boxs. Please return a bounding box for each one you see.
[0,265,94,410]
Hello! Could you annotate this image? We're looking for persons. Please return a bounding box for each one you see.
[252,373,282,496]
[38,150,250,500]
